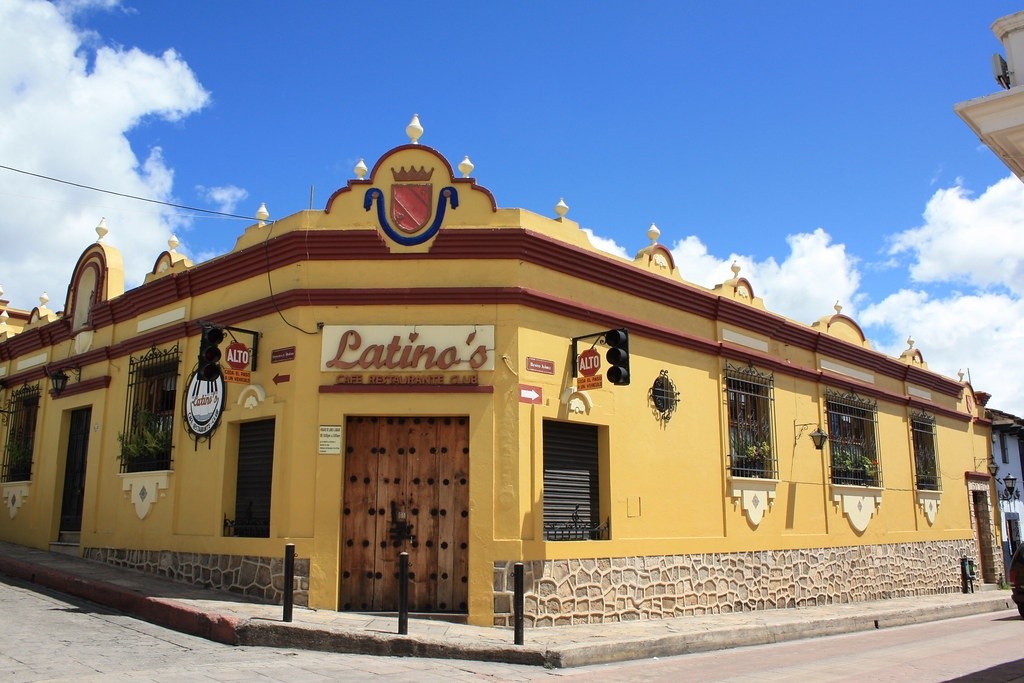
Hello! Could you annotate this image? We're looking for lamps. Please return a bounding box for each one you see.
[974,454,999,476]
[999,473,1021,500]
[793,419,828,450]
[48,365,81,397]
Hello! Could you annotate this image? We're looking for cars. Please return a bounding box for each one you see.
[1010,542,1024,618]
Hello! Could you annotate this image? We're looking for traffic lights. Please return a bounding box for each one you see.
[197,327,224,381]
[606,329,630,385]
